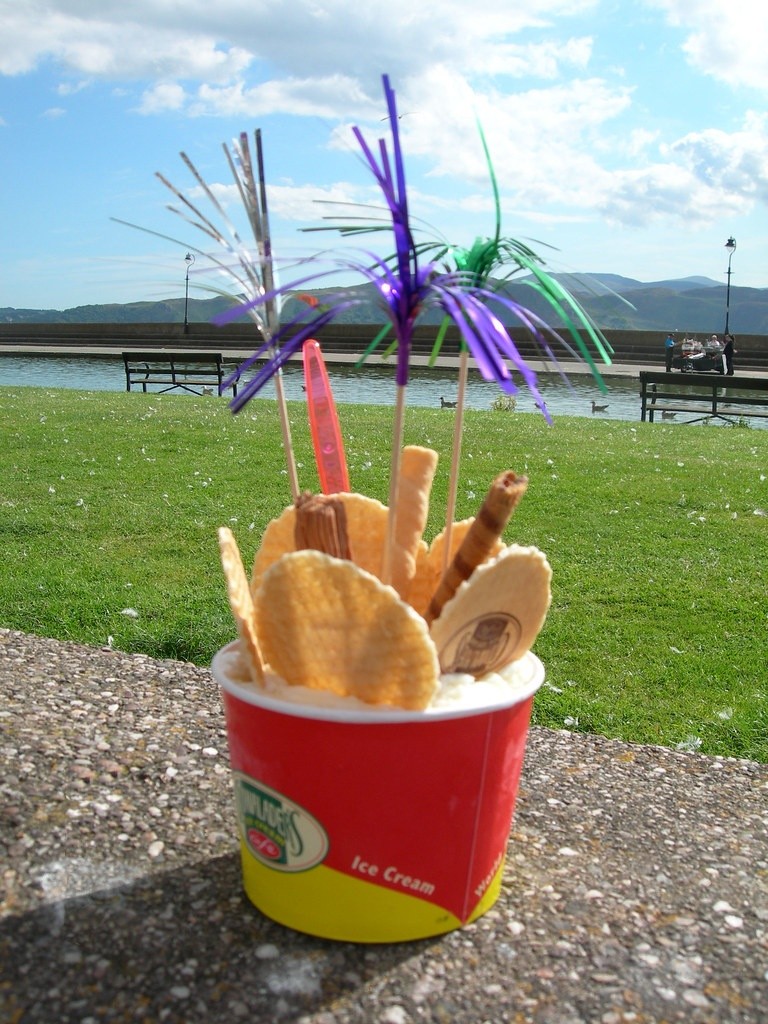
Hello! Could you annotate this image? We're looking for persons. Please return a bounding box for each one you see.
[664,333,676,372]
[708,334,720,346]
[723,334,734,375]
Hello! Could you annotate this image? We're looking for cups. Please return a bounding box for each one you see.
[210,636,547,944]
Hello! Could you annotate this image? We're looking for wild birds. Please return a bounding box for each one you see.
[533,402,548,409]
[661,408,677,419]
[438,397,457,408]
[300,385,305,392]
[242,380,253,387]
[590,400,609,412]
[201,384,214,397]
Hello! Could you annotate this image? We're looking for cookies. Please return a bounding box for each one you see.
[218,442,555,713]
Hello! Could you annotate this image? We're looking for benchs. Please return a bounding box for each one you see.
[640,370,768,424]
[122,351,241,398]
